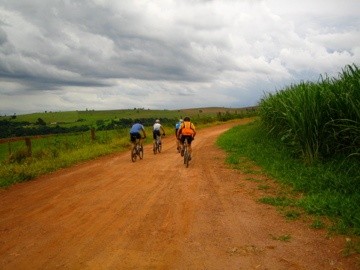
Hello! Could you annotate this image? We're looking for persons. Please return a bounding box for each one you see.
[130,119,146,157]
[152,118,165,149]
[177,117,196,160]
[175,116,185,150]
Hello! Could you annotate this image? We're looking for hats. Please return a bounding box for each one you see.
[156,119,160,123]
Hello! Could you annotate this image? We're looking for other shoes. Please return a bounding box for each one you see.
[181,149,185,156]
